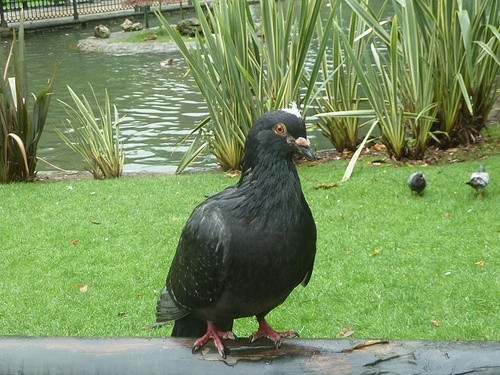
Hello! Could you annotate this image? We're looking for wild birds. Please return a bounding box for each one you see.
[466,163,490,198]
[156,100,317,362]
[407,170,427,197]
